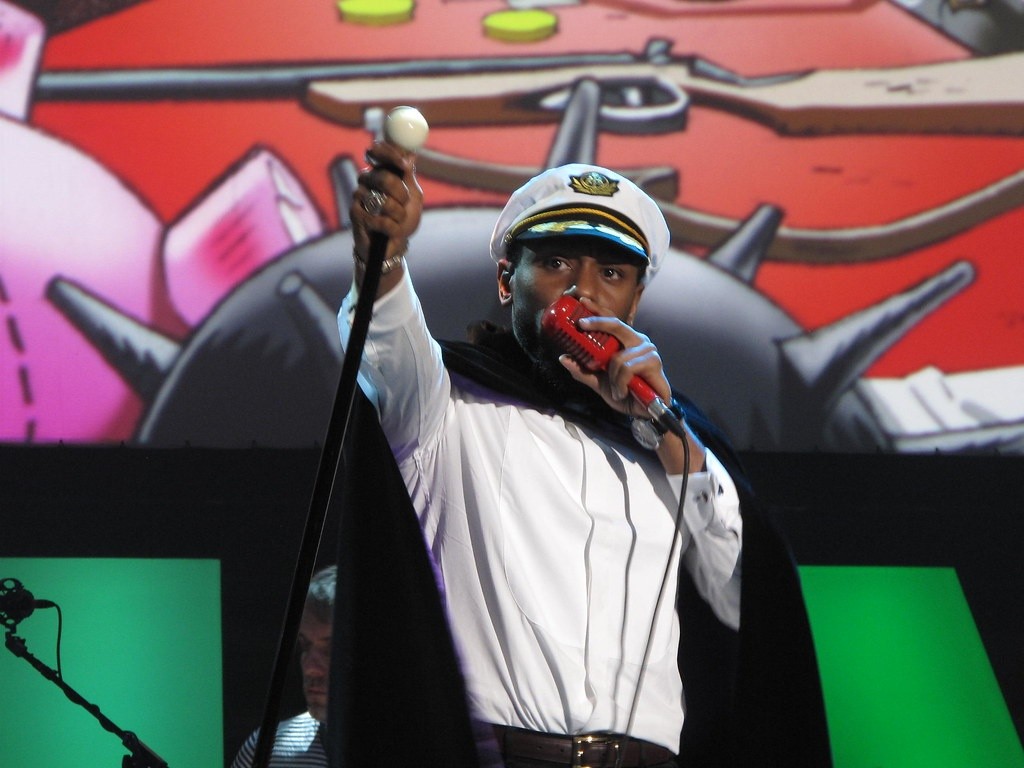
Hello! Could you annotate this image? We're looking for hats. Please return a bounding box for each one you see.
[491,162,669,289]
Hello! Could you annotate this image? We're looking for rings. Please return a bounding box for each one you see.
[363,189,387,216]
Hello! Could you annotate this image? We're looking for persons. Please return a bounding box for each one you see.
[229,566,338,768]
[337,139,743,768]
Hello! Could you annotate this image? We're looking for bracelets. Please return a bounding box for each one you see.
[352,247,401,275]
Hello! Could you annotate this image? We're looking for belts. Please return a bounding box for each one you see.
[474,717,676,768]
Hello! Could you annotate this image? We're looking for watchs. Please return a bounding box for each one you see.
[631,399,685,451]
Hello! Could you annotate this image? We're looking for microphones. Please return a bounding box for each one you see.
[542,296,687,440]
[0,590,59,620]
